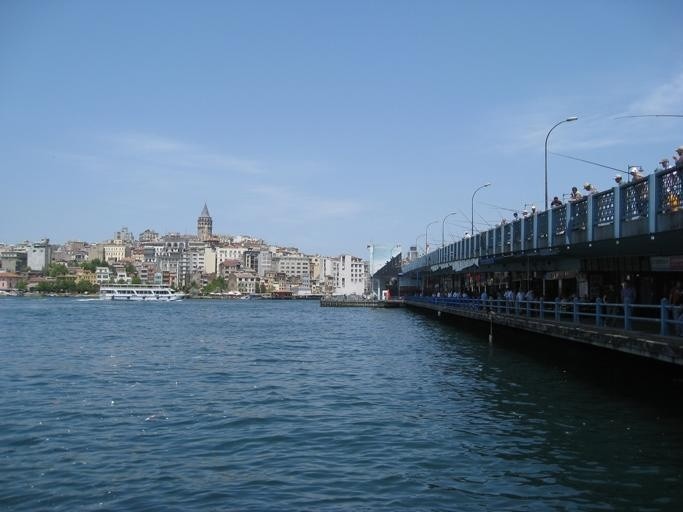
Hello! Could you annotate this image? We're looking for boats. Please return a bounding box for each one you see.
[99,284,185,301]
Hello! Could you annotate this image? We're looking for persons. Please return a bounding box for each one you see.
[320,291,378,302]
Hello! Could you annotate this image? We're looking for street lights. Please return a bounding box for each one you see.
[472,183,491,236]
[544,117,578,211]
[415,213,456,258]
